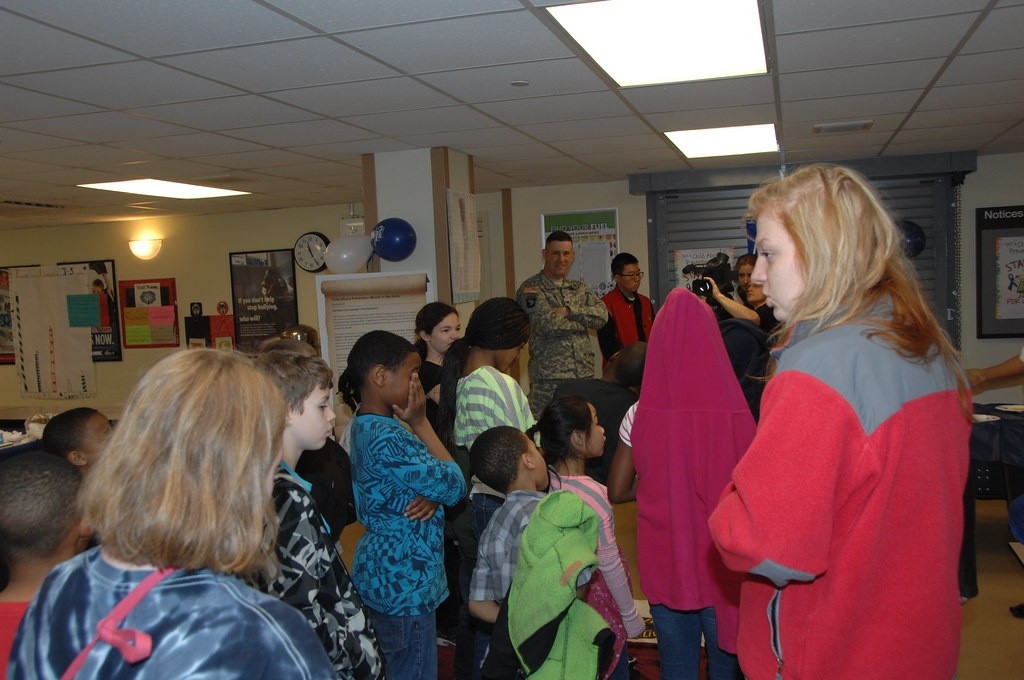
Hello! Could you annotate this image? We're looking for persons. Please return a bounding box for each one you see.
[708,162,976,680]
[347,330,468,680]
[93,273,111,295]
[524,397,647,680]
[552,341,647,486]
[467,426,592,680]
[413,302,462,647]
[717,318,772,425]
[516,232,609,420]
[699,254,779,333]
[0,339,387,680]
[438,296,543,680]
[963,346,1024,618]
[608,287,758,680]
[596,252,656,376]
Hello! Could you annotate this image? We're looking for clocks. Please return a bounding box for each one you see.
[294,232,331,273]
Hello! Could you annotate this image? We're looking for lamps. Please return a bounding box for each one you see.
[128,239,162,259]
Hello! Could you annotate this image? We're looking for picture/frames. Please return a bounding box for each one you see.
[974,204,1024,341]
[229,248,300,362]
[0,259,181,364]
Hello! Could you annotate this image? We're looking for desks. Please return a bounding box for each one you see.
[956,402,1024,597]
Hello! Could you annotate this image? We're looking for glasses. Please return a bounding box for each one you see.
[620,272,643,279]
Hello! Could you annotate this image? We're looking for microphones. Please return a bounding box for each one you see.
[682,265,696,274]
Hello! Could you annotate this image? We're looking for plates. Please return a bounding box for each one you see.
[971,414,1001,421]
[995,404,1024,412]
